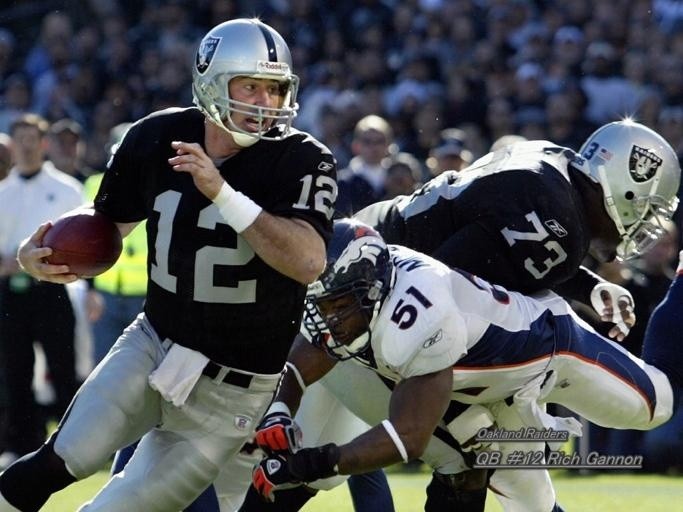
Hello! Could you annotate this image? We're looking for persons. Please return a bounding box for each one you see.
[0,0,683,511]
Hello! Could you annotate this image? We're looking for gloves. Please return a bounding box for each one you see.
[442,399,502,455]
[255,403,305,456]
[250,442,351,503]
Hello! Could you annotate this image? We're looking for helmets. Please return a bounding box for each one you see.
[190,17,298,142]
[300,217,396,363]
[578,120,681,261]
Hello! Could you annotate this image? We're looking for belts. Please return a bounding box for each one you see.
[163,337,256,389]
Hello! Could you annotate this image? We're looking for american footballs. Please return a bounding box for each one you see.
[40,208,123,280]
